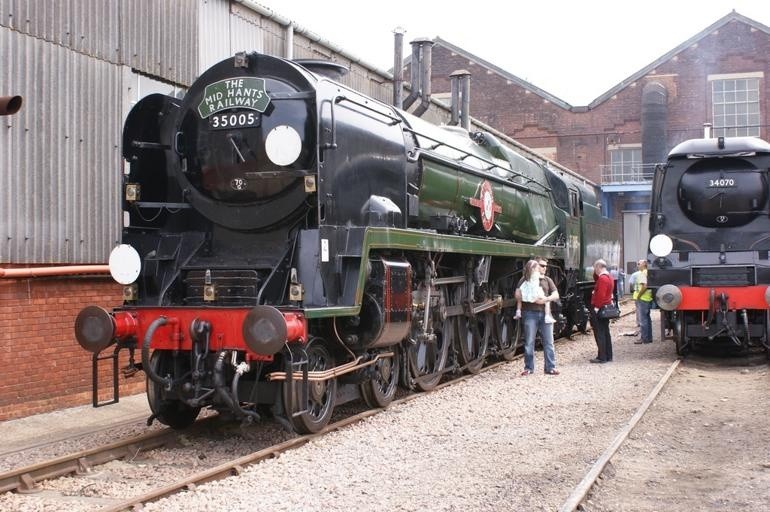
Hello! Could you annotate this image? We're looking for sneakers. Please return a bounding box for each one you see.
[588,356,606,364]
[544,317,557,324]
[543,367,561,376]
[520,369,534,377]
[512,313,522,320]
[633,338,654,345]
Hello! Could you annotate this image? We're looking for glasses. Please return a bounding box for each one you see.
[538,263,549,268]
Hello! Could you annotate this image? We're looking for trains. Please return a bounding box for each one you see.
[644,122,769,356]
[74,48,624,436]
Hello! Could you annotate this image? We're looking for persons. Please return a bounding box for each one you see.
[514,255,560,376]
[629,270,642,327]
[618,268,626,297]
[611,264,618,298]
[512,260,557,324]
[634,259,654,344]
[590,258,615,364]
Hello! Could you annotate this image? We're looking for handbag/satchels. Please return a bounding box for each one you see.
[596,302,621,320]
